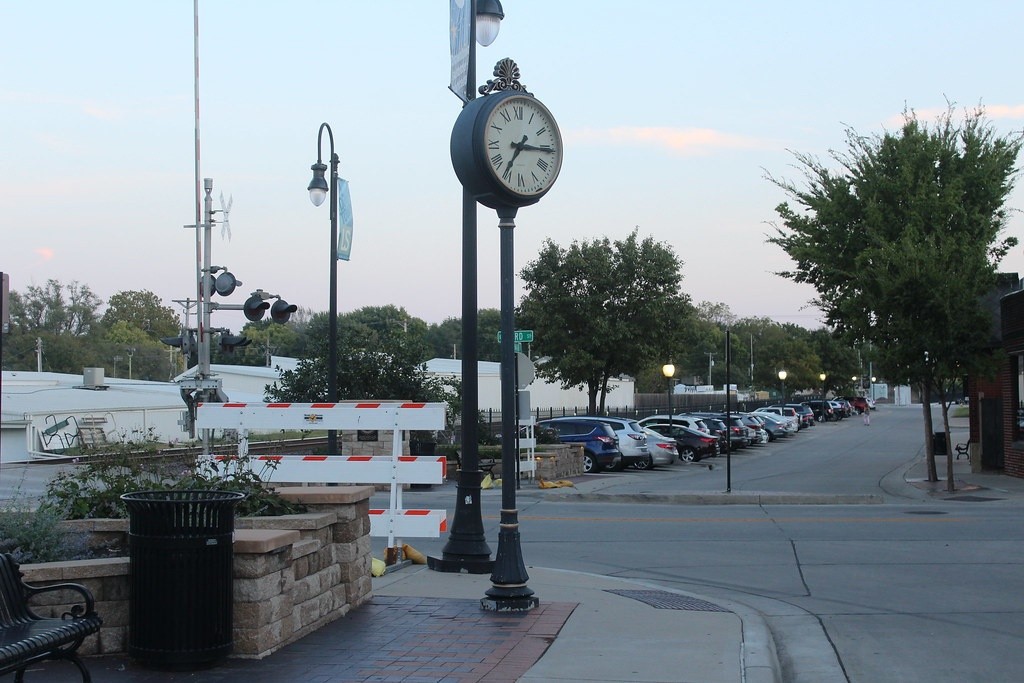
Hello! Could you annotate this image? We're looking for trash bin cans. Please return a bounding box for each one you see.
[409,441,441,489]
[934,431,949,455]
[120,490,247,673]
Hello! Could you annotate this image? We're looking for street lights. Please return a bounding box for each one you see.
[441,0,506,566]
[820,373,827,423]
[852,376,857,396]
[663,364,675,436]
[308,122,340,488]
[778,370,787,416]
[871,377,876,401]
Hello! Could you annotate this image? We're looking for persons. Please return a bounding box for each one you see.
[864,403,870,426]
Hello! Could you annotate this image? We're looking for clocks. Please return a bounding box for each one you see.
[450,91,562,209]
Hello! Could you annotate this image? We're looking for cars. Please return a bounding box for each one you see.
[751,397,876,442]
[702,416,731,454]
[713,412,770,452]
[647,424,721,462]
[517,419,621,473]
[554,416,649,472]
[634,427,679,470]
[639,415,710,435]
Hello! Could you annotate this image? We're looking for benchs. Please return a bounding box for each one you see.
[955,439,970,459]
[0,552,103,683]
[455,449,497,480]
